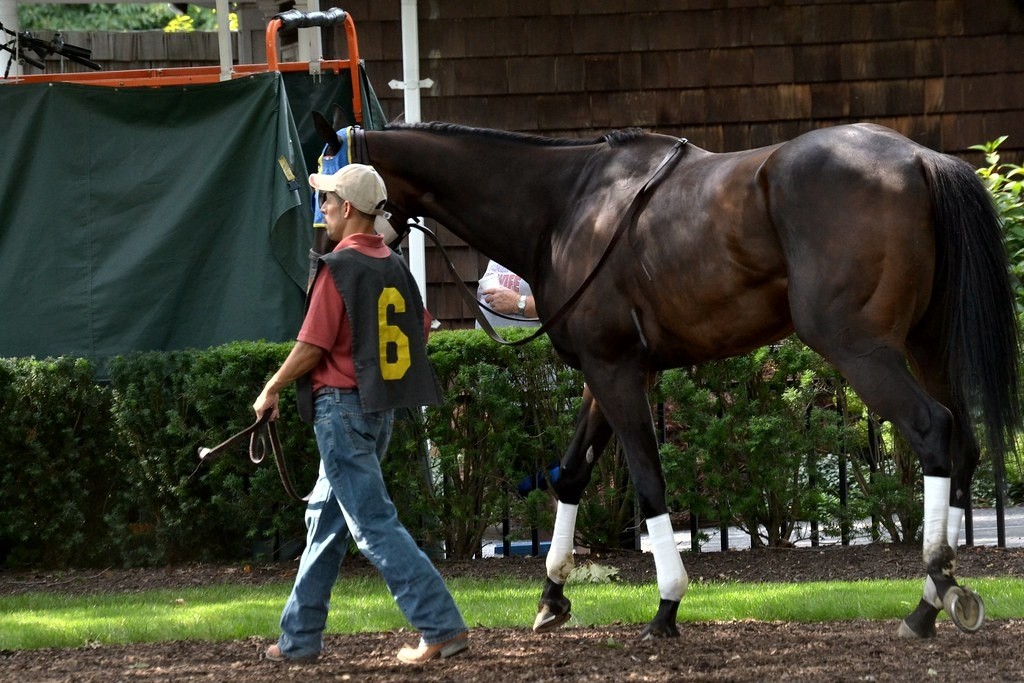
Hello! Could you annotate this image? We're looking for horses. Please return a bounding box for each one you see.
[306,107,1020,637]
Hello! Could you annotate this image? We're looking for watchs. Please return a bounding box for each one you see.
[517,294,527,316]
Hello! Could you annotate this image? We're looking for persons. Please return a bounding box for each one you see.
[254,163,470,666]
[475,259,543,329]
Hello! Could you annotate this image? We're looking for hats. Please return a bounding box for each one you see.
[307,161,393,221]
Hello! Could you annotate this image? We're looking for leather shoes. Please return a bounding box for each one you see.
[396,629,470,665]
[264,644,288,662]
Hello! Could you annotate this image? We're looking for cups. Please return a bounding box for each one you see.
[477,273,500,292]
